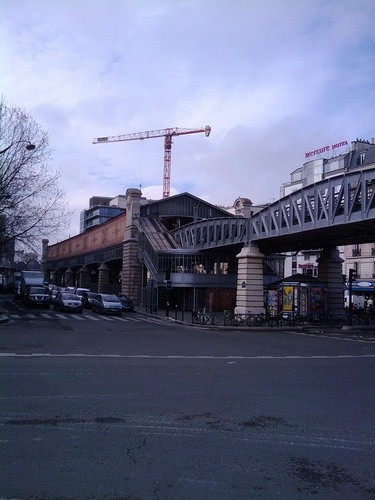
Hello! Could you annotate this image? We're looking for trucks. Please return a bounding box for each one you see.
[13,270,45,300]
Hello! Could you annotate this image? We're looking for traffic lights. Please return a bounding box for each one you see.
[349,268,358,282]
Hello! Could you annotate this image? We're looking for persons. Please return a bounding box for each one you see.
[224,305,232,316]
[364,298,374,310]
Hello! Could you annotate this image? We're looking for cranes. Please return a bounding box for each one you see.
[93,123,211,199]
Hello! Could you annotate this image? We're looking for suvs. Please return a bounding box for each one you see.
[27,286,50,307]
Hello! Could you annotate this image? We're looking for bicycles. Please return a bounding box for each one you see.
[192,309,328,327]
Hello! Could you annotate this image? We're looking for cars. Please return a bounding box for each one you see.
[92,294,123,316]
[53,293,82,313]
[45,283,91,304]
[117,295,135,313]
[81,292,99,308]
